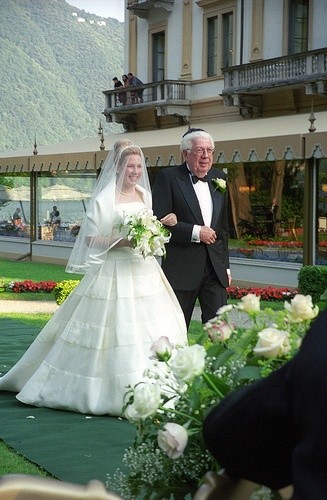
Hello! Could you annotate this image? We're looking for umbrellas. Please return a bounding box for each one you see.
[0,184,91,221]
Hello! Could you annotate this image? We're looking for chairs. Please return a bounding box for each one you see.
[276,215,299,242]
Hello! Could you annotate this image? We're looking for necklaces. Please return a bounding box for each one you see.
[118,187,135,196]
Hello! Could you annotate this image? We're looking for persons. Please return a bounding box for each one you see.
[13,207,21,221]
[50,206,61,224]
[153,131,231,332]
[113,73,144,104]
[0,139,189,417]
[201,305,327,500]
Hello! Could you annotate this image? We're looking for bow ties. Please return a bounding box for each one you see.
[191,174,209,184]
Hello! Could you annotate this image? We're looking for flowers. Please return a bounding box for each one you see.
[7,278,320,500]
[119,210,171,260]
[212,178,227,193]
[229,240,327,258]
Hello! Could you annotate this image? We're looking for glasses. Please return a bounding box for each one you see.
[187,147,215,154]
